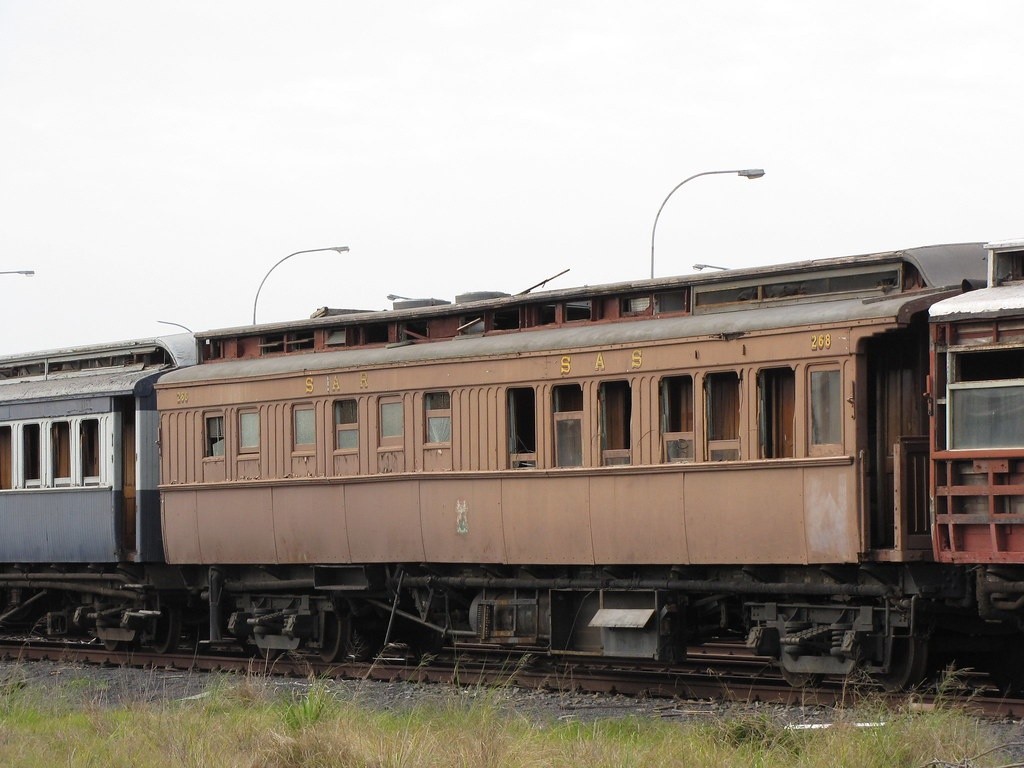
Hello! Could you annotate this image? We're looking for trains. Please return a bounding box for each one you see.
[1,240,1024,700]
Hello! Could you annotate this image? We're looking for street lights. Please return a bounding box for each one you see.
[649,167,768,279]
[252,245,352,325]
